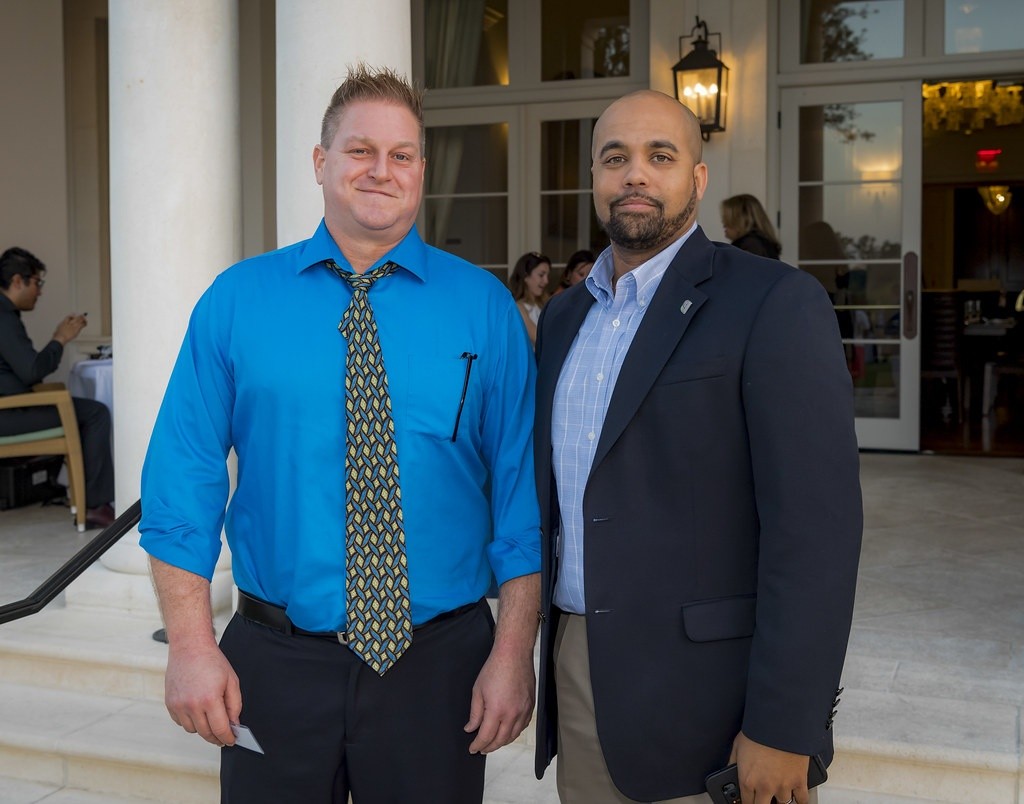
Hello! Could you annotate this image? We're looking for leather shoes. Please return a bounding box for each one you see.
[74,504,115,529]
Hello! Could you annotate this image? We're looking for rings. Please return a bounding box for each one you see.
[786,796,794,804]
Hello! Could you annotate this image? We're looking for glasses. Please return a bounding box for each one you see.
[22,274,45,288]
[527,252,540,262]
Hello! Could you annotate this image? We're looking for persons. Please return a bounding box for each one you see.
[506,88,865,804]
[507,251,551,352]
[139,66,542,804]
[1,245,115,532]
[551,250,597,297]
[717,193,784,263]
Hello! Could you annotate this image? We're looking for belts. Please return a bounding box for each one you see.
[237,589,486,645]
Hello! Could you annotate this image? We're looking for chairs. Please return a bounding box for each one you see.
[0,382,85,533]
[921,365,964,427]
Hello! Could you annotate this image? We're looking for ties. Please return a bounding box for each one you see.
[323,258,413,677]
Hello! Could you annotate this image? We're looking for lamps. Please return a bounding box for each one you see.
[670,15,729,142]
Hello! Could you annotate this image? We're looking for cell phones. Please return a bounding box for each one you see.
[705,754,827,804]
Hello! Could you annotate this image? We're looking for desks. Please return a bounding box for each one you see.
[964,323,1007,335]
[57,358,114,508]
